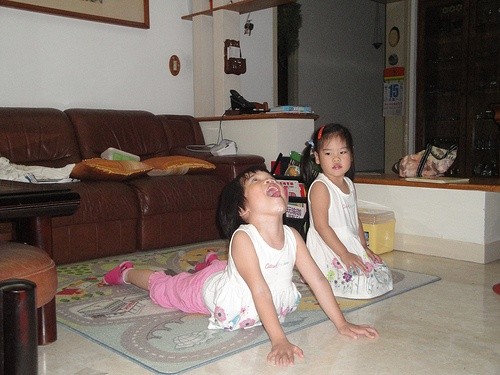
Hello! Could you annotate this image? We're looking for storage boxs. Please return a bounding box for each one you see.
[358,208,395,256]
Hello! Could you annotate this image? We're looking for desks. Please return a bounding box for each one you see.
[0,187,80,346]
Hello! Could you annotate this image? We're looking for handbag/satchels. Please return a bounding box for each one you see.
[399,145,457,178]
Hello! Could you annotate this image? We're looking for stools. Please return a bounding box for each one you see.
[0,241,57,346]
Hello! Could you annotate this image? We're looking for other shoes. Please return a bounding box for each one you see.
[195,251,216,272]
[100,262,133,285]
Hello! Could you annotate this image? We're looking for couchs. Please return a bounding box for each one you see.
[0,106,265,266]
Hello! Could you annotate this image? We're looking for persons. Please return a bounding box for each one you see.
[102,167,380,367]
[298,122,394,300]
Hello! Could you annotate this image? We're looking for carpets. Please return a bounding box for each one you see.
[56,237,442,375]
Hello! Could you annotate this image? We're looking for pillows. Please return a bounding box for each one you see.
[69,157,154,180]
[143,156,217,177]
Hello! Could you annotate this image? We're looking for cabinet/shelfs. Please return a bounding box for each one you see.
[354,174,500,265]
